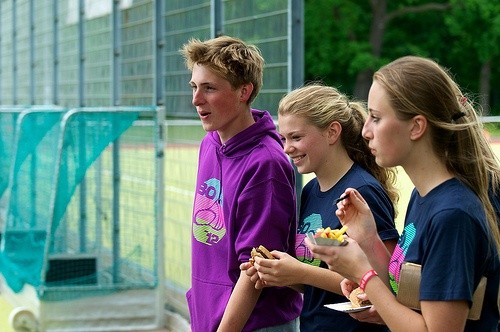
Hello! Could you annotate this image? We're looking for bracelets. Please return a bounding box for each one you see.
[359,269,378,291]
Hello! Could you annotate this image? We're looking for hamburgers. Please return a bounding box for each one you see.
[349,286,373,308]
[249,244,275,267]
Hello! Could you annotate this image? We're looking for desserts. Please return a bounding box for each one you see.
[313,225,349,242]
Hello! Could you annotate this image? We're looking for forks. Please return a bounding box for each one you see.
[333,193,350,205]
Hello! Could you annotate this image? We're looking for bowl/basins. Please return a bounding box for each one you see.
[305,232,348,246]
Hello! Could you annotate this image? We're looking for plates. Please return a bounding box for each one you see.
[324,302,372,312]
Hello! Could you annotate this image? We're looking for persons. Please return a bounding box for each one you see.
[178,35,304,332]
[304,56,500,332]
[240,85,399,331]
